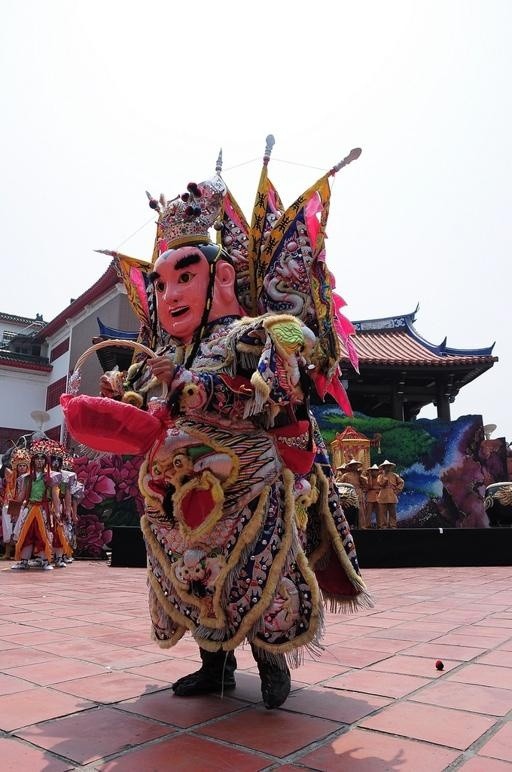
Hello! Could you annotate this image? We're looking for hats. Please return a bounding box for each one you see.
[336,460,397,471]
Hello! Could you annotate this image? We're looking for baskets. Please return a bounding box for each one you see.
[64,338,170,457]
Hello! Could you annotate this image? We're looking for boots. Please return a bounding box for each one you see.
[173,651,236,695]
[251,646,291,710]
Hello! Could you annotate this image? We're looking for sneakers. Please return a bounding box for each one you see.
[11,551,73,570]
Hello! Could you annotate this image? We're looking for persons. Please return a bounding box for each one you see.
[1,443,87,569]
[61,136,368,716]
[339,456,406,528]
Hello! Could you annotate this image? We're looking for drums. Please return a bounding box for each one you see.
[484,482,511,528]
